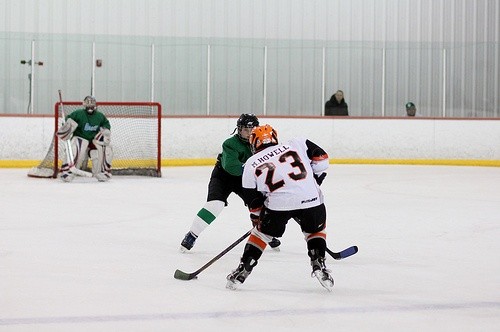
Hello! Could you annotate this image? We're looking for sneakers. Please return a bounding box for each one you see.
[250,212,281,251]
[226,257,258,290]
[308,249,335,293]
[179,230,198,253]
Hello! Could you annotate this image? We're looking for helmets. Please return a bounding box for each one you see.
[237,113,259,130]
[84,96,97,114]
[249,124,278,155]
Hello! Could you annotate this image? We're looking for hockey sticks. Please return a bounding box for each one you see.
[173,226,254,281]
[325,245,359,260]
[57,89,93,177]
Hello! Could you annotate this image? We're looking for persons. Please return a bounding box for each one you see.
[406,102,422,116]
[325,90,349,116]
[225,124,335,291]
[56,96,113,182]
[180,113,281,253]
